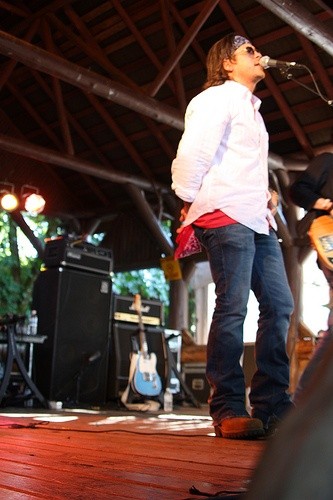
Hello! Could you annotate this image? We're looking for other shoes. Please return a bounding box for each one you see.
[214,416,265,439]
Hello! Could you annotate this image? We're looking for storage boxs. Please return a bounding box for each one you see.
[181,362,214,408]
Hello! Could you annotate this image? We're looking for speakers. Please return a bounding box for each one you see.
[26,270,112,407]
[110,324,167,409]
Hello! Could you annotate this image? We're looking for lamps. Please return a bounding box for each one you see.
[0,181,46,214]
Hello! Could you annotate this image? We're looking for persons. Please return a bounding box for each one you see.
[290,152,333,289]
[171,35,294,438]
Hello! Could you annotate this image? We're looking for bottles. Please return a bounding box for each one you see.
[164,387,173,412]
[27,309,38,335]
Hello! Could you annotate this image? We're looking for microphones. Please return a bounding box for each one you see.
[259,55,305,69]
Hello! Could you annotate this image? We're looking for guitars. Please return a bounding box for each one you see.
[130,294,161,396]
[310,215,333,270]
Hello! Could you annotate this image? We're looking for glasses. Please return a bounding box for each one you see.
[234,47,257,55]
